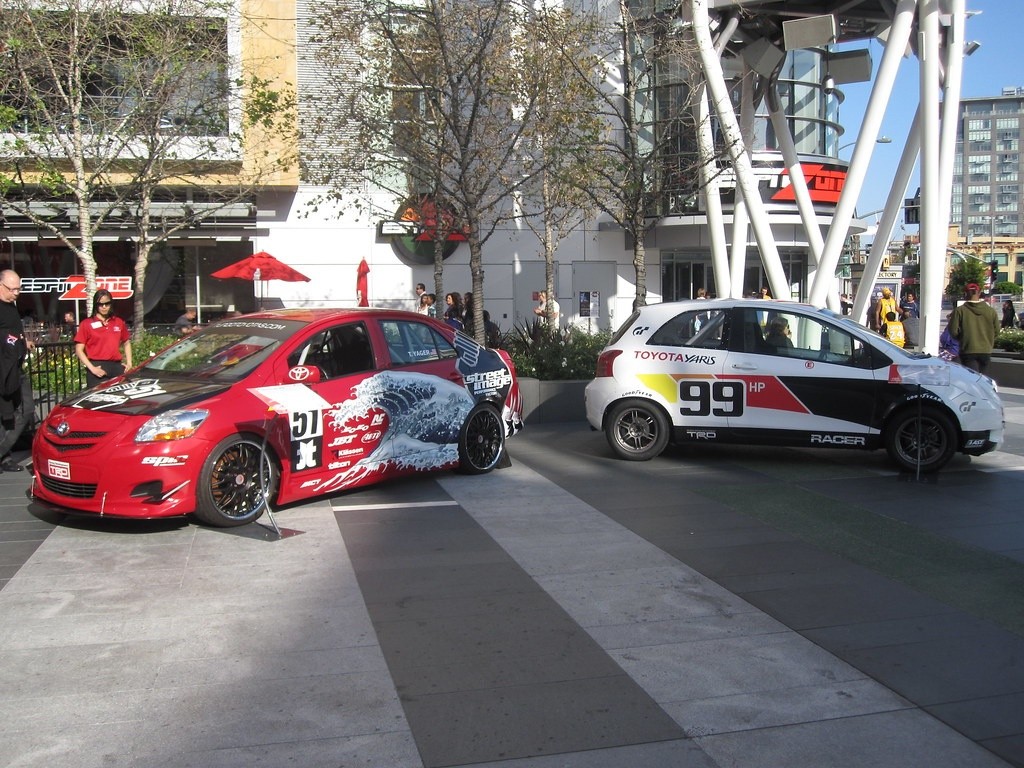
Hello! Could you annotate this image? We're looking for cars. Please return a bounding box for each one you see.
[27,306,527,529]
[584,298,1006,475]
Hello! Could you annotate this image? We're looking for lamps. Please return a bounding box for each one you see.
[964,39,981,57]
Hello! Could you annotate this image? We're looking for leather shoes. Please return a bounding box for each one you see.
[0,460,24,474]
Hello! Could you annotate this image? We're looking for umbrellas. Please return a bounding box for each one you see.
[211,252,312,306]
[354,261,371,307]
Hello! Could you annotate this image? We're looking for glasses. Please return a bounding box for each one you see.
[785,325,789,329]
[416,288,420,290]
[95,301,111,307]
[3,283,20,292]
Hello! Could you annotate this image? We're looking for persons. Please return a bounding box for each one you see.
[63,312,75,334]
[535,290,560,335]
[766,318,794,348]
[841,293,853,315]
[693,288,709,336]
[1001,300,1015,329]
[414,283,502,350]
[866,287,920,347]
[750,287,771,326]
[949,283,1000,373]
[175,309,201,335]
[73,289,133,387]
[0,269,36,471]
[940,324,960,362]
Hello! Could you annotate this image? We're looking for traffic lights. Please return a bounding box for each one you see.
[990,260,999,281]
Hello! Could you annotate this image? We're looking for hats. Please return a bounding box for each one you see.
[964,283,980,293]
[882,288,891,294]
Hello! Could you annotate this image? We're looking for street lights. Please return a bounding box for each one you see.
[982,216,1004,306]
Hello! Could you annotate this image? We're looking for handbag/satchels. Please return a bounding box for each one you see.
[1013,313,1020,323]
